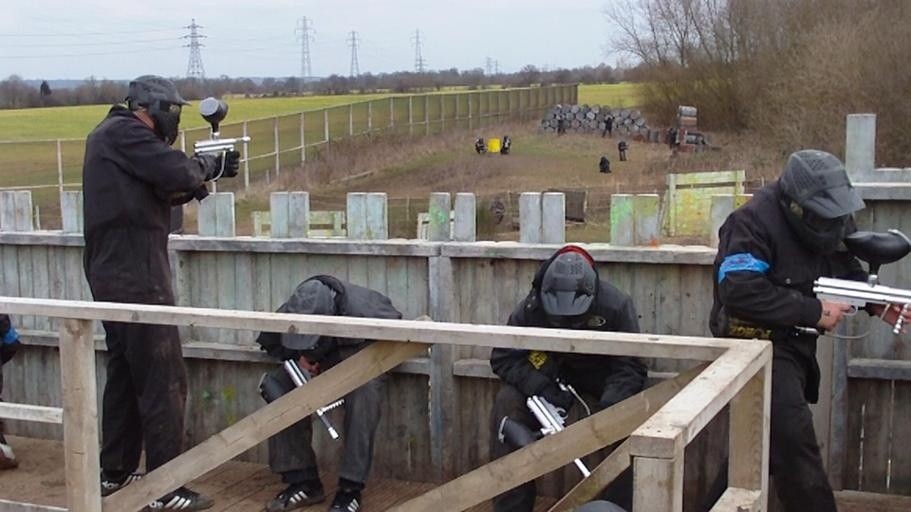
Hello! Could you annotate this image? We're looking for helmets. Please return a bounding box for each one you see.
[280,280,334,350]
[128,74,192,144]
[540,251,598,328]
[780,149,865,250]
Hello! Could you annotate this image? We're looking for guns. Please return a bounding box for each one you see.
[257,359,347,440]
[793,228,911,340]
[193,97,250,180]
[499,377,592,479]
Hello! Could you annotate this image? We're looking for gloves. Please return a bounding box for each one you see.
[538,378,572,417]
[192,150,241,181]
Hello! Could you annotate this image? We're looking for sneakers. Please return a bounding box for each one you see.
[329,488,362,512]
[266,480,325,511]
[149,485,213,512]
[101,468,143,496]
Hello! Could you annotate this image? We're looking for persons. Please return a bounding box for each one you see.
[602,115,615,137]
[490,246,646,508]
[669,127,677,149]
[502,134,510,155]
[82,76,237,512]
[474,138,487,154]
[599,157,611,173]
[618,140,627,161]
[256,278,403,512]
[712,147,875,511]
